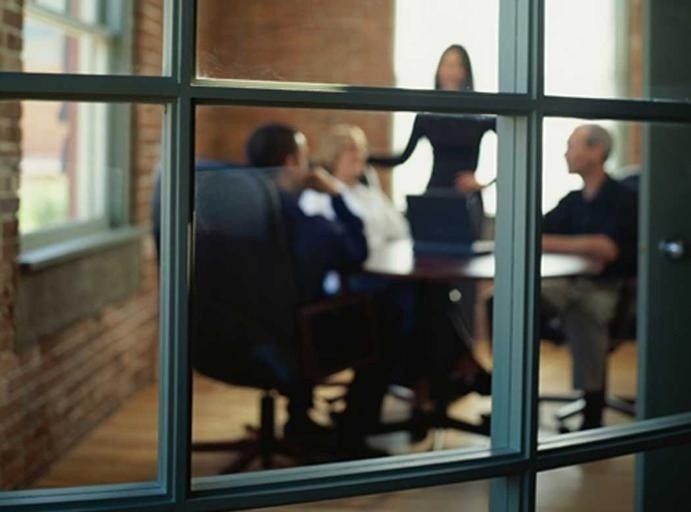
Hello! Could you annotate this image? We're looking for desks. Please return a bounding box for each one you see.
[356,237,605,440]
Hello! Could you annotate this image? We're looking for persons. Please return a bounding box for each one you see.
[244,124,494,464]
[481,124,636,433]
[366,43,500,245]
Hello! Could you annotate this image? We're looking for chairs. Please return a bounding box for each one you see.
[150,166,404,477]
[536,170,640,434]
